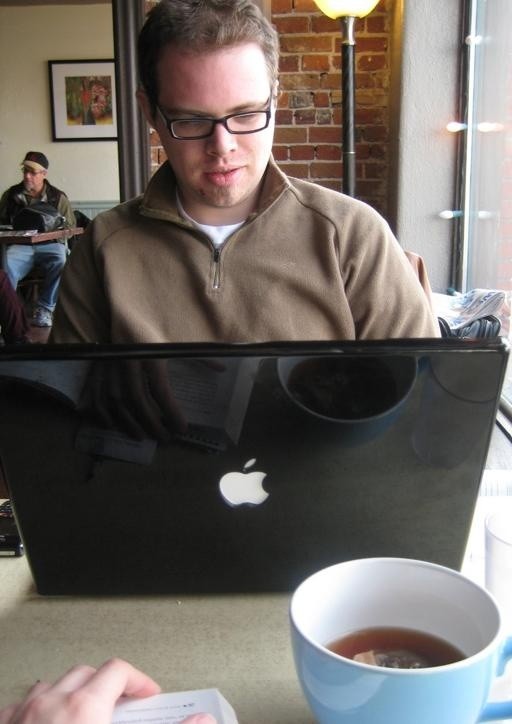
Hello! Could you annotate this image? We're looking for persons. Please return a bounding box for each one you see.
[2,152,76,326]
[45,2,442,347]
[0,659,218,724]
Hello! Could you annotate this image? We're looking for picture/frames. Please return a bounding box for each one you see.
[48,58,118,141]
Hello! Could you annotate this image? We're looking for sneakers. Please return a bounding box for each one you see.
[31,304,51,326]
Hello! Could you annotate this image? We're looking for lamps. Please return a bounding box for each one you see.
[315,0,379,198]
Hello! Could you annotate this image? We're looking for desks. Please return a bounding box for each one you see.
[0,227,84,273]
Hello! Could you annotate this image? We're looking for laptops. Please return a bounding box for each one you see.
[0,335,511,596]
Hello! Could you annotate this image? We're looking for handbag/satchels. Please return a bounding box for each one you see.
[13,203,62,232]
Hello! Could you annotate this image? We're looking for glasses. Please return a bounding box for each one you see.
[155,88,272,139]
[21,167,41,175]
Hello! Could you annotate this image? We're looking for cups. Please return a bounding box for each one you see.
[408,353,499,472]
[288,555,506,723]
[269,353,417,443]
[482,509,512,635]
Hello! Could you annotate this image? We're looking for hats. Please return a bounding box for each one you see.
[22,152,49,171]
